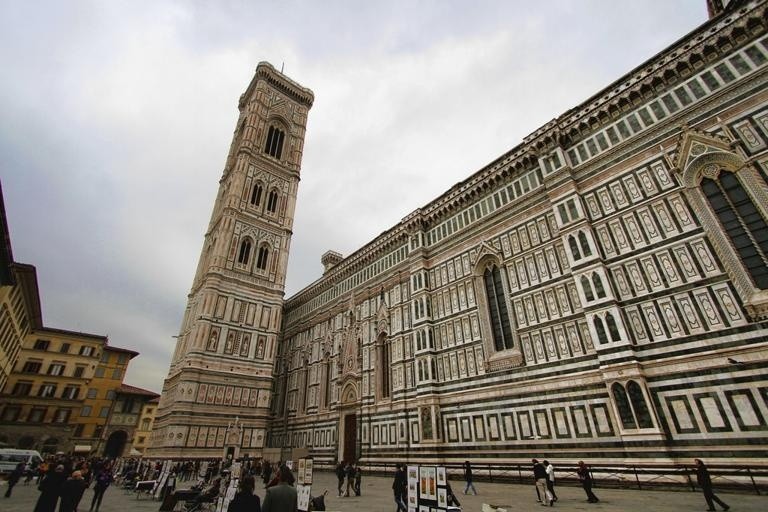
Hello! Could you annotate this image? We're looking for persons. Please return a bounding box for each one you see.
[4,455,297,512]
[392,462,407,512]
[462,461,476,496]
[534,460,553,507]
[532,459,546,502]
[695,459,730,512]
[336,461,362,497]
[401,465,408,506]
[543,461,558,501]
[577,461,599,503]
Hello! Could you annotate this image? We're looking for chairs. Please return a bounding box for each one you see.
[182,493,221,512]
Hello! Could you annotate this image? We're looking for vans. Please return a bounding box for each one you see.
[0,448,44,474]
